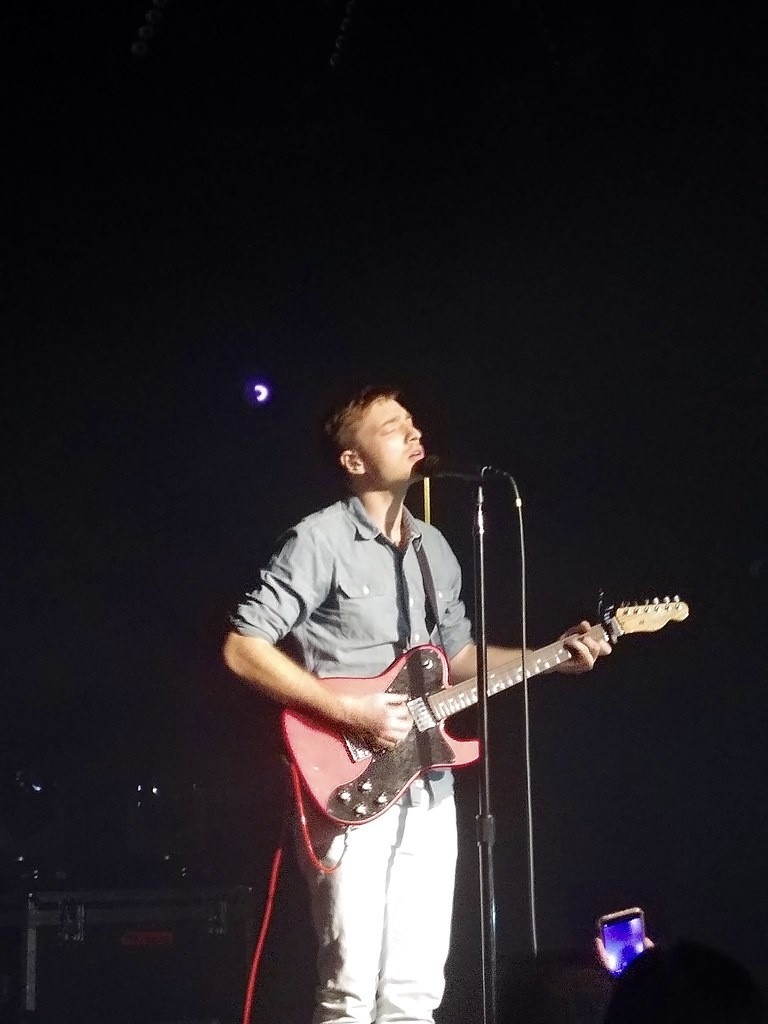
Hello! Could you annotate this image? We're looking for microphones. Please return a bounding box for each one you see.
[419,454,495,488]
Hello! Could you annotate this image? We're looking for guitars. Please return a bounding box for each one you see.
[280,595,690,826]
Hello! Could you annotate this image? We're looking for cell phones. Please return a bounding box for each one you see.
[598,906,646,977]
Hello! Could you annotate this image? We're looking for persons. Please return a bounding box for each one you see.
[591,934,768,1024]
[220,384,612,1024]
